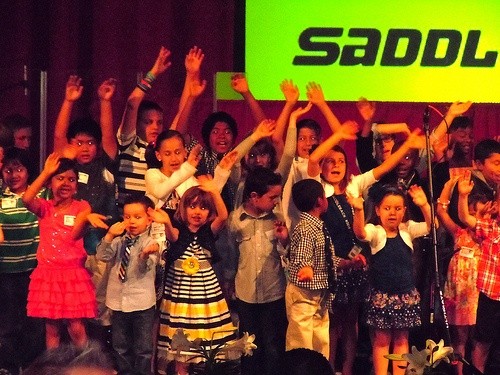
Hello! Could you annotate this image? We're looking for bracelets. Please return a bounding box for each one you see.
[148,73,154,79]
[141,79,151,88]
[437,198,450,208]
[137,81,148,93]
[147,74,153,80]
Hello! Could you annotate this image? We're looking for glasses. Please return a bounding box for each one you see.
[376,134,395,146]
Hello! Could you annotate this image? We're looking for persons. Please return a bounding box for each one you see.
[286,178,364,360]
[0,112,53,375]
[435,168,488,374]
[282,120,431,374]
[347,184,439,373]
[147,173,242,373]
[96,195,160,372]
[228,169,294,359]
[21,152,110,348]
[47,47,500,255]
[457,168,500,375]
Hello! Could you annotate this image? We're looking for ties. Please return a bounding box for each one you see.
[119,236,139,283]
